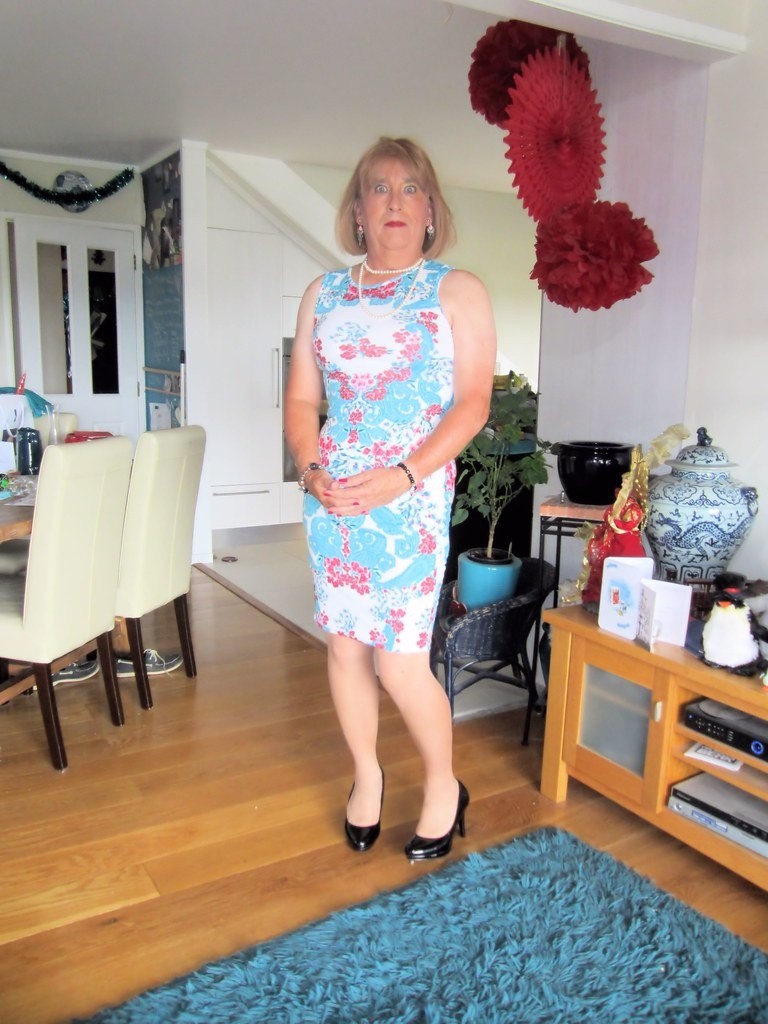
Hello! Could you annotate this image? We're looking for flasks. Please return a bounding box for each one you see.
[17,428,43,474]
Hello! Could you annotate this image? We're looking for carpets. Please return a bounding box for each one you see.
[82,826,768,1024]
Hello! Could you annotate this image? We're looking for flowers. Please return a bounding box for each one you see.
[505,46,607,225]
[468,18,592,129]
[527,201,661,314]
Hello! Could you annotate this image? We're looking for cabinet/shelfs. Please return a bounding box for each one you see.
[539,602,768,890]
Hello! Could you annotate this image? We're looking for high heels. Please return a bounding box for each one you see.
[405,779,470,859]
[344,768,385,851]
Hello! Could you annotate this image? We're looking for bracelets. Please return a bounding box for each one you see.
[298,462,324,494]
[397,463,417,493]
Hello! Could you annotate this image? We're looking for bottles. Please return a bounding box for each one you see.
[45,403,64,447]
[645,428,758,583]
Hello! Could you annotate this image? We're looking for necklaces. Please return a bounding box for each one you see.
[358,256,426,319]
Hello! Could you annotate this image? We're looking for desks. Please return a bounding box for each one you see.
[523,491,617,746]
[0,472,131,700]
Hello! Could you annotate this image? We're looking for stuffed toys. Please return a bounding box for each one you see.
[702,591,768,678]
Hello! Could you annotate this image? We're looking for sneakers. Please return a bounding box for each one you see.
[32,660,100,690]
[115,649,183,677]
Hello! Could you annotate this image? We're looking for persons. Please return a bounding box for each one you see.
[281,135,497,860]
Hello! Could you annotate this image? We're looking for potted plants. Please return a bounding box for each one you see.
[448,371,552,610]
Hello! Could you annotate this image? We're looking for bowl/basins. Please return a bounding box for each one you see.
[550,441,635,504]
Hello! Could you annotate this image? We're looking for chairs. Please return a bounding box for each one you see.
[0,424,208,771]
[431,557,560,745]
